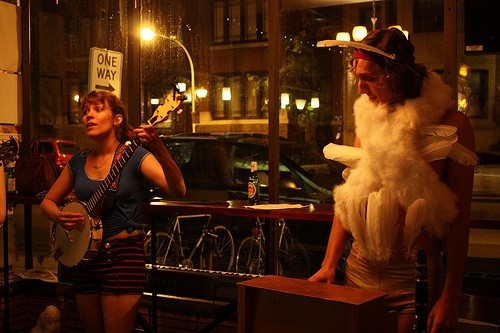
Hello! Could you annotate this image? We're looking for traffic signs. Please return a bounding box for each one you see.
[88,46,123,100]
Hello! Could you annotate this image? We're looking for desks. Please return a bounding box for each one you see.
[142,197,332,333]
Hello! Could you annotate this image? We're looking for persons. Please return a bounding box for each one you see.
[42,91,186,333]
[308,28,474,333]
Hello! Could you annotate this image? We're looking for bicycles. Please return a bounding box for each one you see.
[235,216,311,280]
[141,214,236,273]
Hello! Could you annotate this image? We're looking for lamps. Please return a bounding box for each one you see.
[336,0,409,47]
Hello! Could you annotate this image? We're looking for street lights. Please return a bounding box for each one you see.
[142,28,196,134]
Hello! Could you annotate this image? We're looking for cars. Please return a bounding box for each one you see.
[31,139,81,176]
[472,151,500,199]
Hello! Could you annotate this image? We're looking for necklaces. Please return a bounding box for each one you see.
[93,148,114,170]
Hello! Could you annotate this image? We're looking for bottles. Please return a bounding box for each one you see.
[247,161,260,200]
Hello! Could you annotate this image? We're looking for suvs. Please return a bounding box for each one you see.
[138,131,346,209]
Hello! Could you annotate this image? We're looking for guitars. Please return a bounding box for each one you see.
[51,89,188,268]
